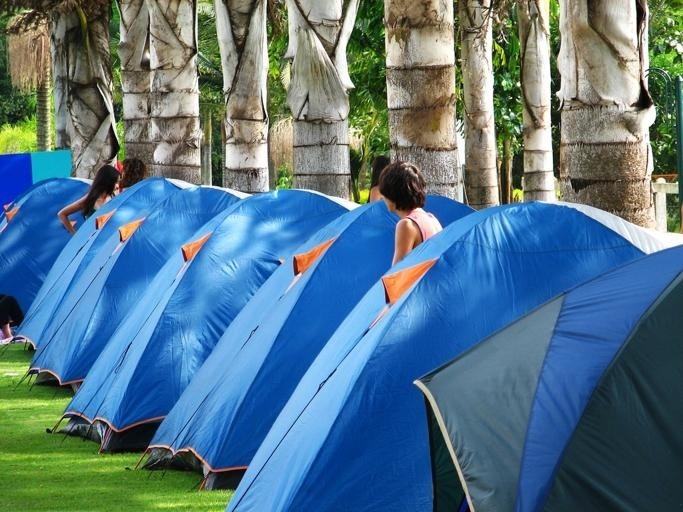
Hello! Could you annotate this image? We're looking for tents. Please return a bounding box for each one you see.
[413,245,682,512]
[134,196,478,489]
[1,178,249,395]
[49,189,363,456]
[224,201,682,512]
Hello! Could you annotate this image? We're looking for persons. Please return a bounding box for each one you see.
[369,156,392,203]
[56,164,120,235]
[376,162,443,267]
[116,159,145,189]
